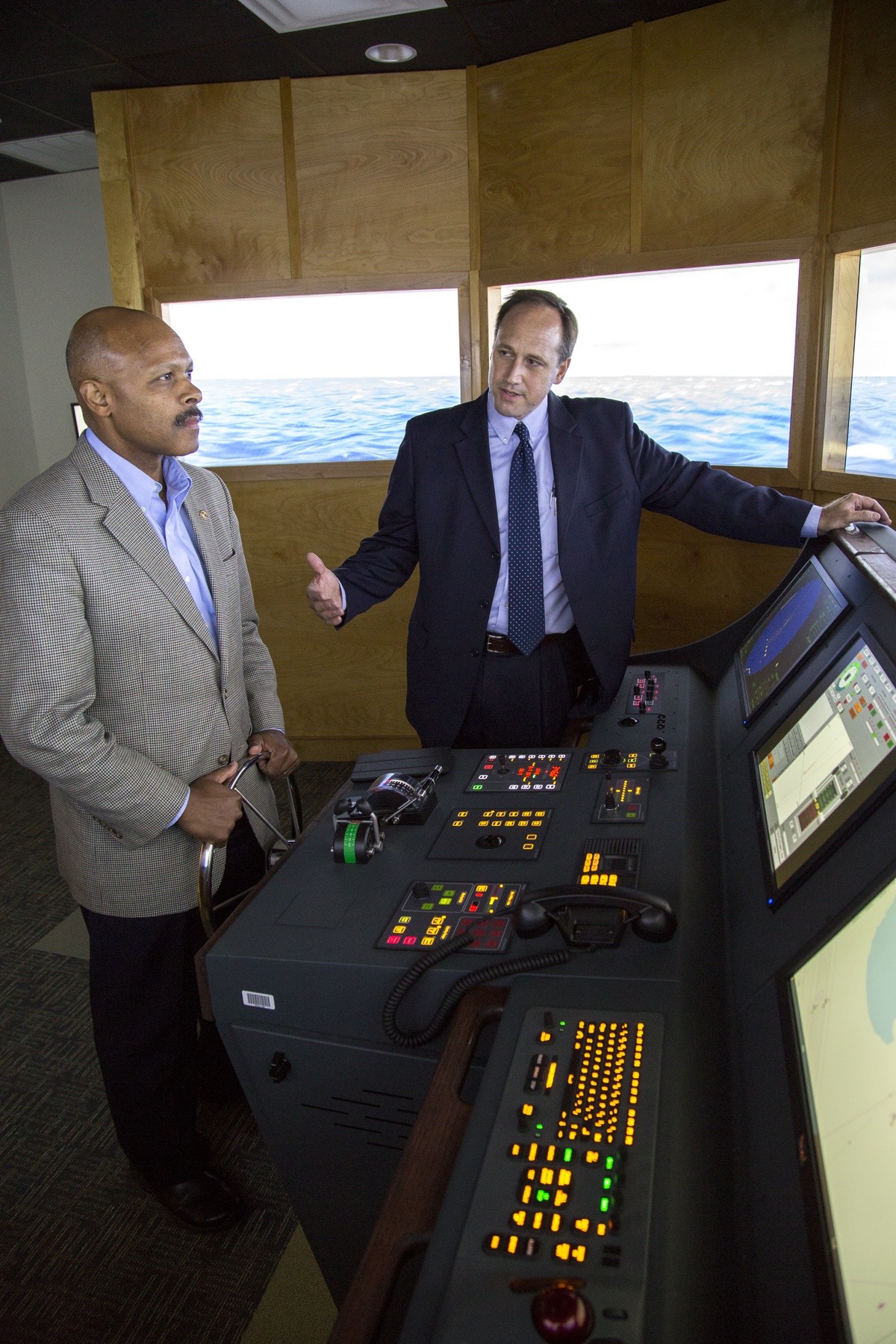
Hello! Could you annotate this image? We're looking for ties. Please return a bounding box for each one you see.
[508,422,545,657]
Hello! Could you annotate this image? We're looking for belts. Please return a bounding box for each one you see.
[486,625,577,659]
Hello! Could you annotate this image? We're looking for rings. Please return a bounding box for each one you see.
[283,771,288,775]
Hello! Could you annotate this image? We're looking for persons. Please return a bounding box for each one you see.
[305,287,893,755]
[3,304,311,1224]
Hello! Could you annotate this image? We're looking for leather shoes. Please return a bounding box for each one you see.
[130,1168,240,1232]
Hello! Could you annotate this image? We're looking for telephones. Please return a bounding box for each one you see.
[513,884,677,942]
